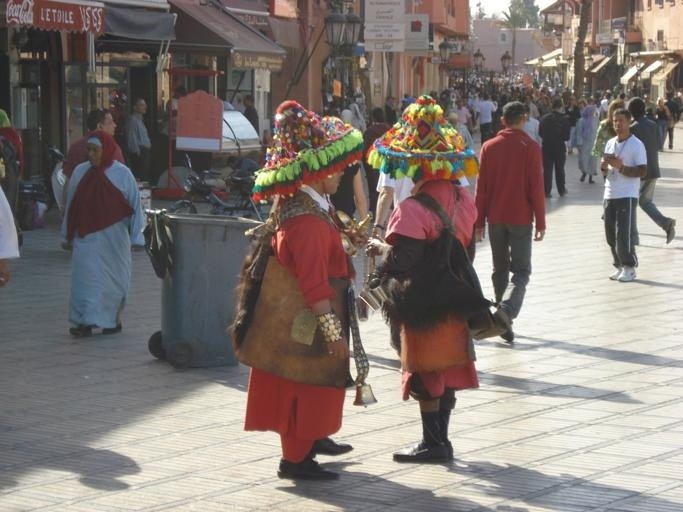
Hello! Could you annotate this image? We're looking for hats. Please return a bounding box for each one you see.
[366,93,483,181]
[249,99,366,203]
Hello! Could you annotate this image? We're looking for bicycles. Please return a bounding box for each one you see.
[164,148,274,221]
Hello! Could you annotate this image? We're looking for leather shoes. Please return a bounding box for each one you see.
[309,438,353,457]
[278,456,341,481]
[662,218,676,244]
[392,439,455,463]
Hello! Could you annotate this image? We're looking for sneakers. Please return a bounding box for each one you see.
[102,322,122,334]
[69,324,93,336]
[545,175,594,198]
[492,306,514,342]
[609,266,635,282]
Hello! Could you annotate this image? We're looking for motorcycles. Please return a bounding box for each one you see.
[17,141,68,230]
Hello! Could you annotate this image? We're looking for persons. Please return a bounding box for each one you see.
[0,108,26,287]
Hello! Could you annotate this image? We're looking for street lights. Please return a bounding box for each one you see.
[471,47,512,89]
[321,2,363,112]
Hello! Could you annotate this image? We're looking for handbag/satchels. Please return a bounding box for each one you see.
[388,191,492,326]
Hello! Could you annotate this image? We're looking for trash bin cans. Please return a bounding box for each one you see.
[144,208,264,369]
